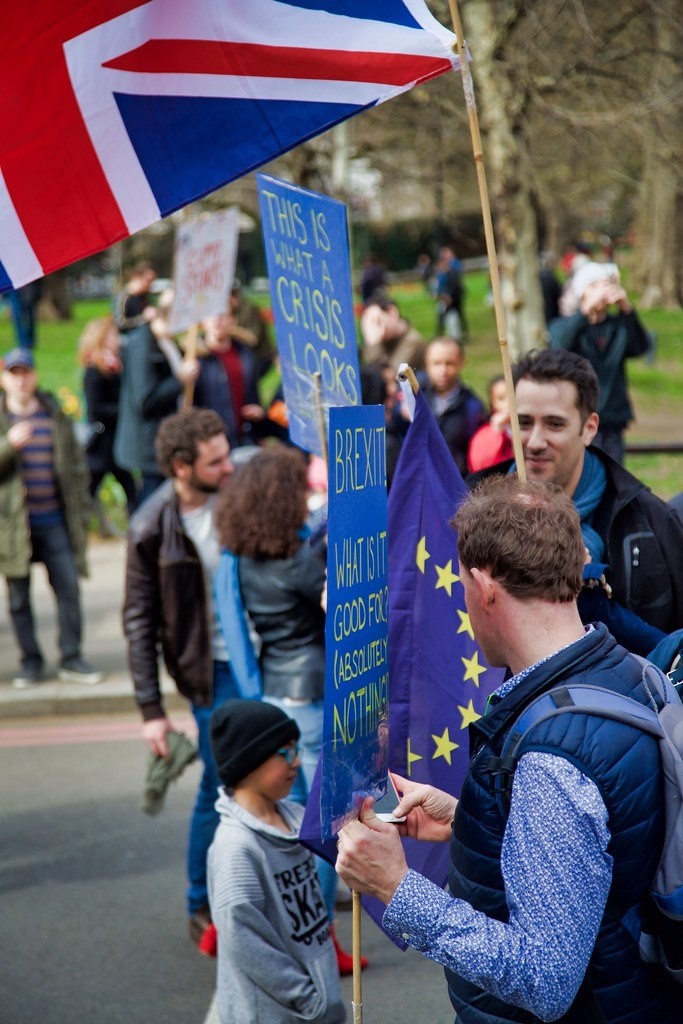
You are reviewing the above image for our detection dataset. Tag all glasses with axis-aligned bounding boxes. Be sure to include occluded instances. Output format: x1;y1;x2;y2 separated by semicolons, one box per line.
275;744;306;765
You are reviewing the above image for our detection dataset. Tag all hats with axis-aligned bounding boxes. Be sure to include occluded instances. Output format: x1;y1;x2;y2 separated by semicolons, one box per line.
5;347;34;372
210;701;302;788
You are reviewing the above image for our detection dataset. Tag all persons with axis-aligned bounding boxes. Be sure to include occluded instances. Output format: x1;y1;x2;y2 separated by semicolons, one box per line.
205;700;348;1023
0;248;683;972
334;476;682;1023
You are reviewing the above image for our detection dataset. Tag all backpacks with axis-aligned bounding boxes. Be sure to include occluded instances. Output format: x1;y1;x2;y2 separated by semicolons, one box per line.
486;651;683;985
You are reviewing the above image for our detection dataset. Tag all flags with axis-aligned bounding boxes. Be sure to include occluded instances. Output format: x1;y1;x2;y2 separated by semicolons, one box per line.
0;1;472;290
298;389;506;953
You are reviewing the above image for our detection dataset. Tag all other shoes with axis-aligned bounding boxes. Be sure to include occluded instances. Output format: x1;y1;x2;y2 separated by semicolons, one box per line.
189;909;212;944
12;659;44;689
57;653;100;683
329;924;368;974
